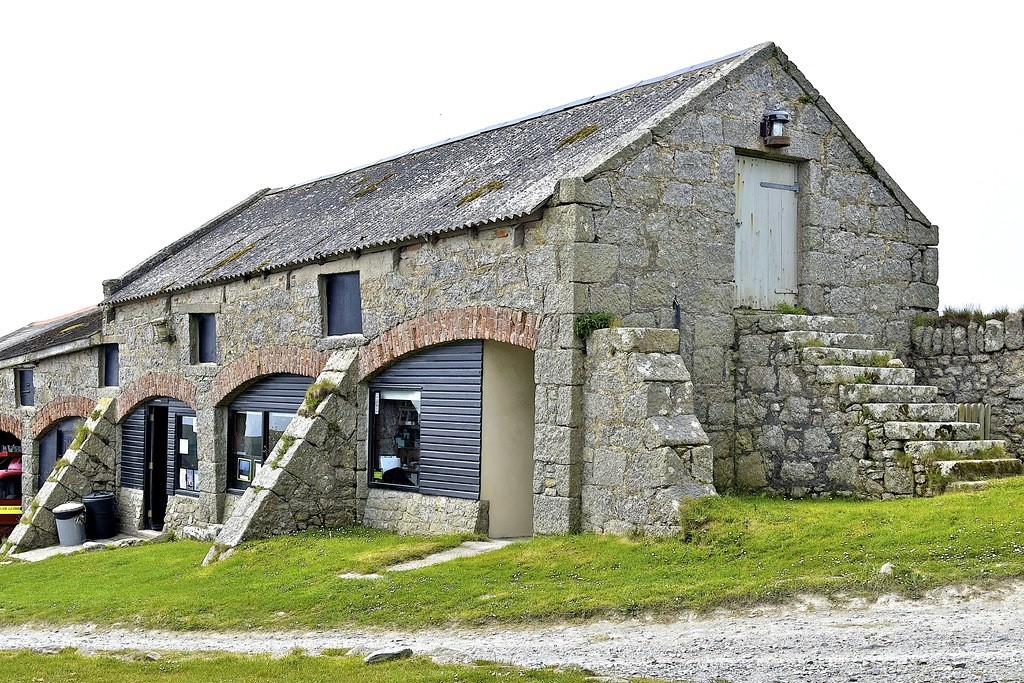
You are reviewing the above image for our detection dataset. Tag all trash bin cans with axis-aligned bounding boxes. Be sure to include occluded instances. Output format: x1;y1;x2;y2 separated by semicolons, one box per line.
51;502;86;547
81;491;117;541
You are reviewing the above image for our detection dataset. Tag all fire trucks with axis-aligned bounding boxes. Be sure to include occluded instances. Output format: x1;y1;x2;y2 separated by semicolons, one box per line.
0;446;24;541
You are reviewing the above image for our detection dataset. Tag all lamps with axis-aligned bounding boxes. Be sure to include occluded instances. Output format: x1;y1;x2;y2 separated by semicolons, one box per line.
763;111;791;146
150;318;176;344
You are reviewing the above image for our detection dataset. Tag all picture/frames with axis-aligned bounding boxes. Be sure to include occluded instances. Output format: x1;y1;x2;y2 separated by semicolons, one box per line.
237;457;252;482
